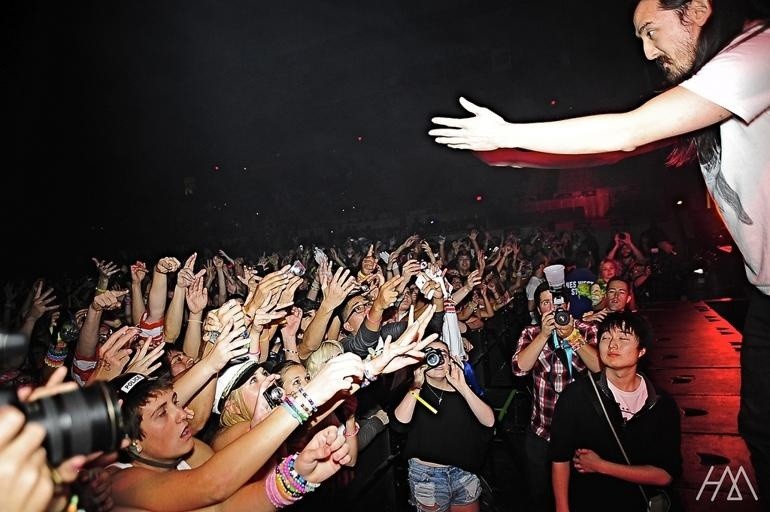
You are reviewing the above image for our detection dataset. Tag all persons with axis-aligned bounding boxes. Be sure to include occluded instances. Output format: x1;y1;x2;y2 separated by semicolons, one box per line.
429;0;770;512
1;215;728;512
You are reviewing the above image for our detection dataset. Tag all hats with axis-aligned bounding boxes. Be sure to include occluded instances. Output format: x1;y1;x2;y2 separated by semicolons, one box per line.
212;353;261;415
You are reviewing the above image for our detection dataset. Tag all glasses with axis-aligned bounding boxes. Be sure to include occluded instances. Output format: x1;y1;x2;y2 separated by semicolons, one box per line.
345;301;373;322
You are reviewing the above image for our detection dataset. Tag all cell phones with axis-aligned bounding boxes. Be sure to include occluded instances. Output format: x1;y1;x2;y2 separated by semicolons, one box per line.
253;265;264;283
414;275;435;300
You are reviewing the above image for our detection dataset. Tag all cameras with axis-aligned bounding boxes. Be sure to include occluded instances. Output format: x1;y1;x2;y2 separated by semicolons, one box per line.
413;259;429;271
0;330;120;467
618;231;627;240
285;260;306;280
550;283;572;330
263;384;286;409
359;279;372;294
420;347;444;371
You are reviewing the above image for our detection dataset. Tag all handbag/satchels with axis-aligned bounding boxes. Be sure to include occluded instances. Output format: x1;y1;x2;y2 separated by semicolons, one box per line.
645;490;672;512
